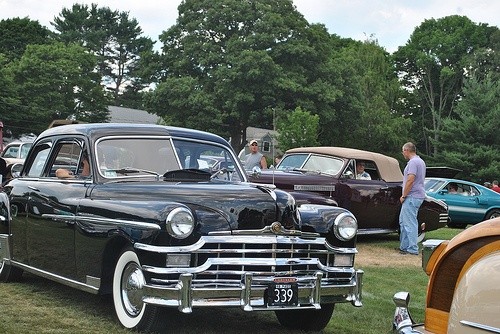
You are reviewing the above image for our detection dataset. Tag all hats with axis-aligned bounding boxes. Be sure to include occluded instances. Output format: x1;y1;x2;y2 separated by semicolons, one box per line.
249;140;258;145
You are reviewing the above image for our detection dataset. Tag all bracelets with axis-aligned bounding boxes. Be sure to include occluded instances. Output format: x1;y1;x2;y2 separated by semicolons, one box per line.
402;195;407;198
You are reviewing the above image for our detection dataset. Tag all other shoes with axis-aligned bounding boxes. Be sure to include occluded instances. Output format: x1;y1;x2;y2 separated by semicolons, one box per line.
394;247;407;254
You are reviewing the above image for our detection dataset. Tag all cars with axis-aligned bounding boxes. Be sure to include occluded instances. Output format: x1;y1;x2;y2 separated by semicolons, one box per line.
388;218;497;331
186;153;253;184
421;175;497;226
0;120;366;332
3;141;78;174
239;144;449;242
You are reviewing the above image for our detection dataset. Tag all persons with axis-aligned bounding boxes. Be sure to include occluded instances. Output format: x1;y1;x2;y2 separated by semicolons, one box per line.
398;142;426;255
449;182;458;193
484;180;500;193
55;148;104;178
356;162;372;180
244;139;267;171
274;154;283;165
